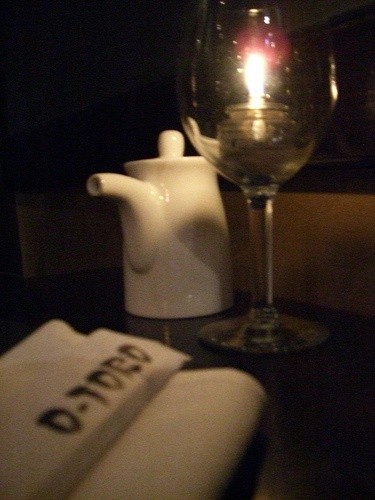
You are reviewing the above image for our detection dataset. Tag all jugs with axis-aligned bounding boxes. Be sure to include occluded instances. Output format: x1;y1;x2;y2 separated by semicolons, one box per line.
82;128;236;319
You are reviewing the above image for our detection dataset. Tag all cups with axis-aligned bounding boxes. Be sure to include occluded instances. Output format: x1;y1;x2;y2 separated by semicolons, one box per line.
180;0;338;353
